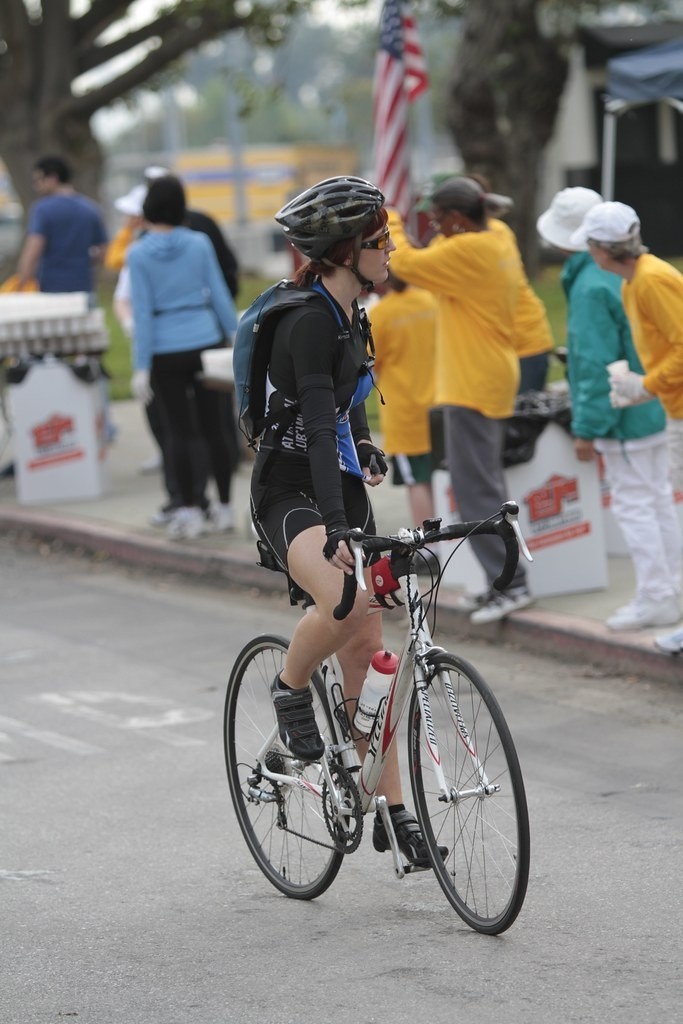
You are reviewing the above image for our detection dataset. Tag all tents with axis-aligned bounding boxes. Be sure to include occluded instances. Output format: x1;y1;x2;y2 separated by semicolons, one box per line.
601;41;683;201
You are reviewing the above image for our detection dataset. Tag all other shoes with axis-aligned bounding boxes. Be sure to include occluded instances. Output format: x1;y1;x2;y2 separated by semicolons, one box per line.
167;508;203;541
208;504;234;532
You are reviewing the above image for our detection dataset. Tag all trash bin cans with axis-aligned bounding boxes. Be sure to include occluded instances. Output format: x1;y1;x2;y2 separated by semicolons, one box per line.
427;389;610;599
2;354;110;504
591;451;683;559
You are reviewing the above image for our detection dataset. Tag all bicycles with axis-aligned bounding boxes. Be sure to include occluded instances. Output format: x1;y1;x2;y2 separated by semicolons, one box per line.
222;500;531;937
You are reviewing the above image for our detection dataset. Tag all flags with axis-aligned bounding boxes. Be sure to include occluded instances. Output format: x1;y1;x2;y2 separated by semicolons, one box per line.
374;0;429;230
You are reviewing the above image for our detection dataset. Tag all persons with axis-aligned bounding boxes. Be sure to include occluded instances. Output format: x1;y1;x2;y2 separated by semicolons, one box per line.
365;270;441;578
383;178;531;622
104;168;238;537
535;186;680;628
251;175;449;867
12;155;115;442
574;202;683;653
413;173;551;393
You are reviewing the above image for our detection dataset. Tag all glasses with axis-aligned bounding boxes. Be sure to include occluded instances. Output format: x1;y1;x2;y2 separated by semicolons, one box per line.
361;231;390;249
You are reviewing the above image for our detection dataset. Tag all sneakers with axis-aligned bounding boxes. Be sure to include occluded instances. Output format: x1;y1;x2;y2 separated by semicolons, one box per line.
372;811;449;867
604;595;681;628
469;590;532;623
270;668;325;759
654;627;683;654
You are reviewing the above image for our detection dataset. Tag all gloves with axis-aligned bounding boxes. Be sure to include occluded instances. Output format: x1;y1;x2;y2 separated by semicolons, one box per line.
607;372;654;408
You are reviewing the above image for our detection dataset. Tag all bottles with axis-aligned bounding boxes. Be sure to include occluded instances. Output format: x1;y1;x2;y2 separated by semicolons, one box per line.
352;650;398;736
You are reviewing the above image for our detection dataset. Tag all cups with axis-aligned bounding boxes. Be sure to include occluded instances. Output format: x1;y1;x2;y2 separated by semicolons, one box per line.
607;360;629;378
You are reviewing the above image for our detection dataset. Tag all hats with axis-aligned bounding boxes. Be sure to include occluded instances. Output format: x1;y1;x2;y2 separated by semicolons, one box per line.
571;202;640;246
536;187;605;252
142;178;184;225
115;185;149;216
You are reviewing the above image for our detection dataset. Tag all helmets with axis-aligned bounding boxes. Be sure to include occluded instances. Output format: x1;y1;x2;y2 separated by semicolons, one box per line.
273;176;385;259
